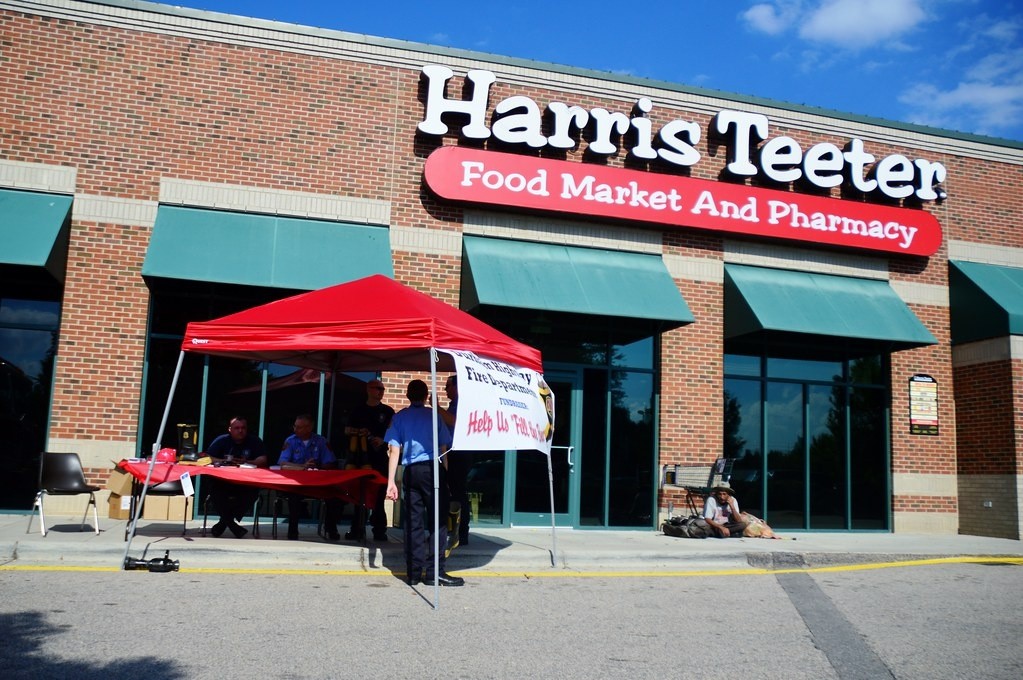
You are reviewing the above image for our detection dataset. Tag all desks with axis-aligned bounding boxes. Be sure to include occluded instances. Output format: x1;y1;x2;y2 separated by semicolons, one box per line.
114;458;390;547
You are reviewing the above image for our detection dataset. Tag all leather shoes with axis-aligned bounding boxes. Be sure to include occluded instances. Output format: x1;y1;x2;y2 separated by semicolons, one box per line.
426;571;464;586
408;576;420;585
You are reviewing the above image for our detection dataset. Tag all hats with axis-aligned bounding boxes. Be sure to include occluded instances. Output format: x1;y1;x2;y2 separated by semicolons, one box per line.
711;481;735;495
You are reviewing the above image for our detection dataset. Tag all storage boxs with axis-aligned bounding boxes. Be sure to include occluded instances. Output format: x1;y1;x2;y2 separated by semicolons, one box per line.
169;495;194;521
108;492;131;520
107;458;133;496
144;495;169;520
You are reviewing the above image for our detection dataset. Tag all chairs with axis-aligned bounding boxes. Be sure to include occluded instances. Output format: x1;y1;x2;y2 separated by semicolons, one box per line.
26;451;101;537
202;494;260;539
272;496;326;540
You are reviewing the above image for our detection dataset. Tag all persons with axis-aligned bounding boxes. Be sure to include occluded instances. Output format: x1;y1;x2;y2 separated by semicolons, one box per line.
345;380;395;543
428;375;470;545
206;416;268;537
277;415;341;540
383;380;463;587
703;481;741;537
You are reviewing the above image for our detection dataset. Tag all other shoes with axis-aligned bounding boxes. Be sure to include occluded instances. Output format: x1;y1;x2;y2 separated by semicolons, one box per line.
373;533;387;541
230;522;248;538
211;521;227;535
323;523;341;540
345;530;366;540
288;523;298;540
460;537;469;544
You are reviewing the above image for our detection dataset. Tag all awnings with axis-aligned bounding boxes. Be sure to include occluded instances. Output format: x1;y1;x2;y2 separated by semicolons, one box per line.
460;235;696;323
0;187;74;283
141;205;395;290
724;262;939;345
948;259;1023;347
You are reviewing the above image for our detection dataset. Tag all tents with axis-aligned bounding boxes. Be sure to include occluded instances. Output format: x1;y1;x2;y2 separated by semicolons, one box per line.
120;274;557;609
233;367;367;395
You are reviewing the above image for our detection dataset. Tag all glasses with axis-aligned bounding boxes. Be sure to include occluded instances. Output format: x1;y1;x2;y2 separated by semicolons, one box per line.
371;386;385;391
